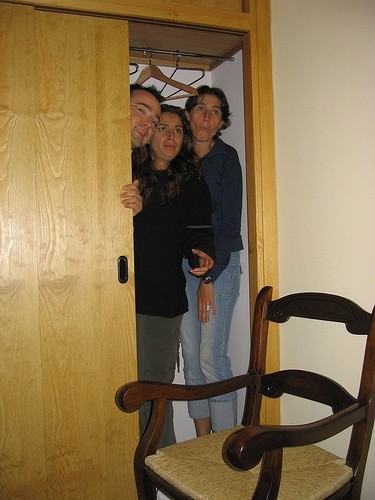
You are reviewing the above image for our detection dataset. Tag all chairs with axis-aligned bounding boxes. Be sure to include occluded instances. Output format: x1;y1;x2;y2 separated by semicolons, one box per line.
114;286;375;500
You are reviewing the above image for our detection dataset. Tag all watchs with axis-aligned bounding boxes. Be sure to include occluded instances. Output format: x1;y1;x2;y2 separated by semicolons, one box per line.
202;274;214;284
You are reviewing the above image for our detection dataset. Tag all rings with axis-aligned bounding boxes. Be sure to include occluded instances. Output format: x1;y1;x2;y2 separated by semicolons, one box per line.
206;304;211;311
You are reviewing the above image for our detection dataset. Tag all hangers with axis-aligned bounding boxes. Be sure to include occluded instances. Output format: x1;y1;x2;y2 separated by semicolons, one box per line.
135;46;198;102
130;62;139;76
161;51;205;98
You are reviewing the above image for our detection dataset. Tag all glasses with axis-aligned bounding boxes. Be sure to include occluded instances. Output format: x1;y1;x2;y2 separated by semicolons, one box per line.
130;102;160;129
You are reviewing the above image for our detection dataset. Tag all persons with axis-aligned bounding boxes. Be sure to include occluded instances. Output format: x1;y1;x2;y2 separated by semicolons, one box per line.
120;83;167;218
178;86;243;438
134;104;216;449
